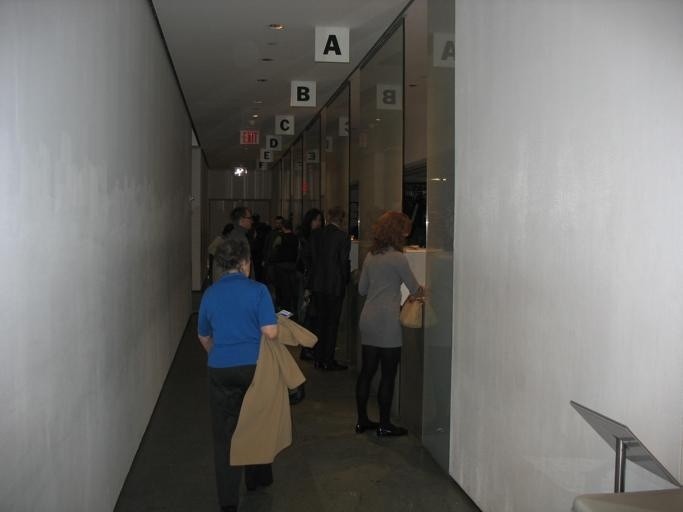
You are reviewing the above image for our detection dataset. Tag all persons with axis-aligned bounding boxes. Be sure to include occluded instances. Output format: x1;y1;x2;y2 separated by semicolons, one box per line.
206;206;351;371
197;235;281;511
354;210;424;438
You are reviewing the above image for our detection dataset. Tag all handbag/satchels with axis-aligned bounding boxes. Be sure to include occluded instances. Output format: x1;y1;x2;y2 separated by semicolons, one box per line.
400;295;422;329
201;275;212;292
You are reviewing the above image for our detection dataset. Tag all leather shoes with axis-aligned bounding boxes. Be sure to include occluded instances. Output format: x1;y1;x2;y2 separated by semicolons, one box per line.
299;352;313;361
356;422;378;433
314;360;347;371
378;424;407;437
